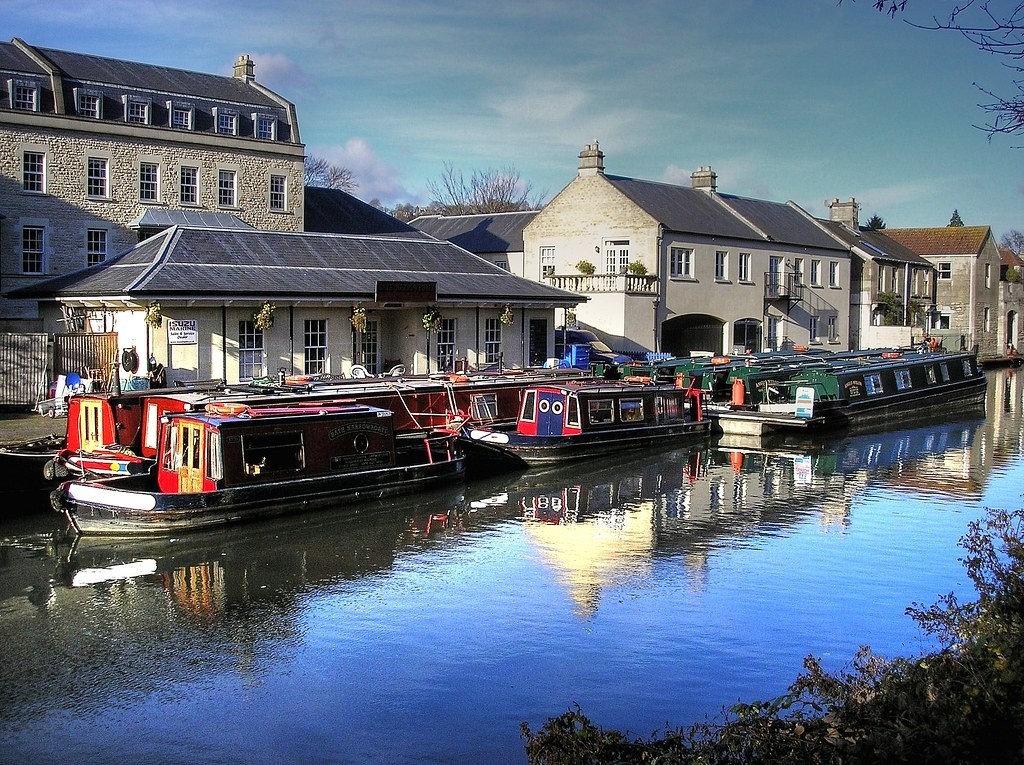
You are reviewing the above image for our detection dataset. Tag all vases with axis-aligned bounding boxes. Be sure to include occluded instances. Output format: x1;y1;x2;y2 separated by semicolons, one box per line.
620;267;628;274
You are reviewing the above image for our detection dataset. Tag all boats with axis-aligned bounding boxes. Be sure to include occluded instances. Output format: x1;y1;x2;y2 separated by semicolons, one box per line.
590;351;986;431
457;377;714;469
44;384;472;536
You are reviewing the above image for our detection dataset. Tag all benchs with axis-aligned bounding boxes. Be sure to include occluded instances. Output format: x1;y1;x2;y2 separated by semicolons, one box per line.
310;372;346;381
172;378;225;386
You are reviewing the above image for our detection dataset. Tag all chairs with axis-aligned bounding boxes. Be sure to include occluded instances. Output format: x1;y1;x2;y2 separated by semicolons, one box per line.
350;364;375;379
382;364;406;377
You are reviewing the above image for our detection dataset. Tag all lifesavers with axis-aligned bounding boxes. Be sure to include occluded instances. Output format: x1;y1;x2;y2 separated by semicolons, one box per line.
450;375;469;383
204;401;247;413
882;352;899;358
624;374;651;382
285;375;309;385
793;345;808;351
711;357;729;364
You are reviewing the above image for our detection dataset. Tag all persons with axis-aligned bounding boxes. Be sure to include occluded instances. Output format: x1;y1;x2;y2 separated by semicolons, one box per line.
926;338;938;350
746;339;753;353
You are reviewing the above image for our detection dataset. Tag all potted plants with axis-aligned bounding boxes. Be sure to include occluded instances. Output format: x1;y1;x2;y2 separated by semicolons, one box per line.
421;307;443;333
497;304;515;325
575;260;596;274
628;260;649;275
252;301;277;331
143;302;162;329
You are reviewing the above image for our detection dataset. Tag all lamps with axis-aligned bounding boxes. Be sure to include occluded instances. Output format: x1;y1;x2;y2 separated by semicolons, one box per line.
787;259;790;265
595;246;599;253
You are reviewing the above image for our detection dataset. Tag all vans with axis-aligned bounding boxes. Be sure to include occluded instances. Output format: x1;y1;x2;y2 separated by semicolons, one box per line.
555;328;633;368
